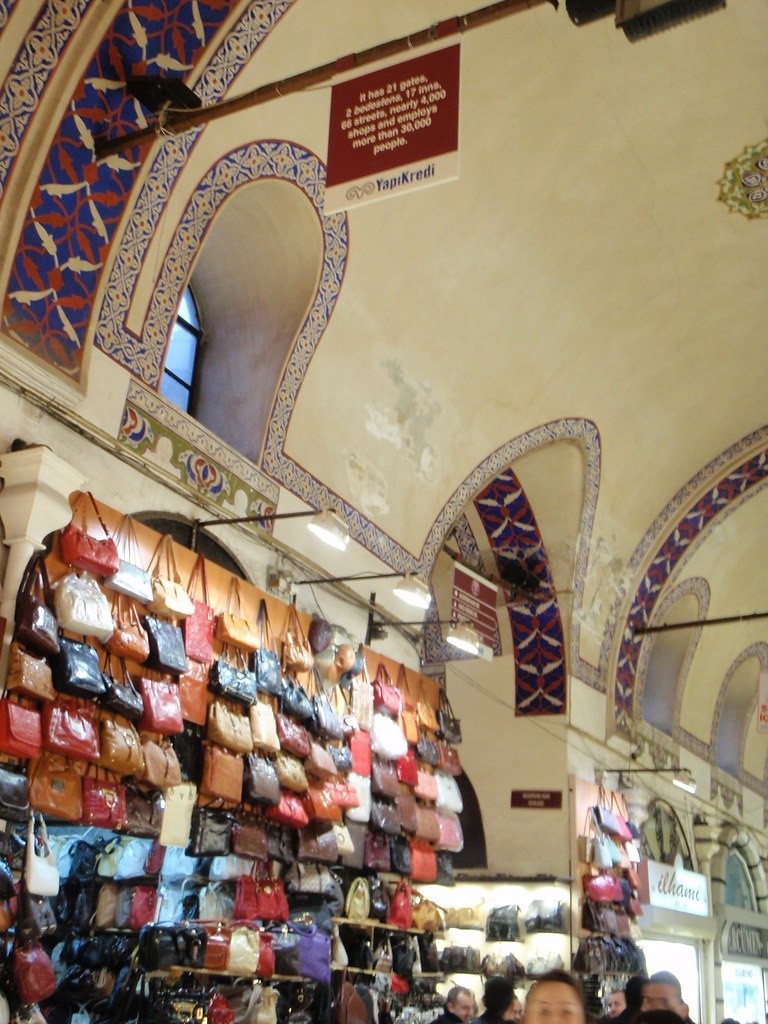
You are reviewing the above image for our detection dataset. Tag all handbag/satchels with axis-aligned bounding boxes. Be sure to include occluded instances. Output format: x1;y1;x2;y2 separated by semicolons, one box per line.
0;488;563;1024
577;785;643;978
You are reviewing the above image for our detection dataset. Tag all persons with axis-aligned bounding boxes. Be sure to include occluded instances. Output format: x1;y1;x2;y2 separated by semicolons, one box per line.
720;1018;760;1024
523;968;586;1024
428;987;475;1024
596;970;696;1024
471;974;523;1024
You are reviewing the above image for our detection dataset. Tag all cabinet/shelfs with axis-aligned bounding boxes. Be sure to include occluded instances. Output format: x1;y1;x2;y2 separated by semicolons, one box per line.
332;915;445;978
412;872;572;980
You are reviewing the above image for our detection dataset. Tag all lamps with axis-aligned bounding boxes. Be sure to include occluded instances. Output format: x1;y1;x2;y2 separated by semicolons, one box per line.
605;767;697;794
197;508;350;552
292;569;431;610
364;593;479;656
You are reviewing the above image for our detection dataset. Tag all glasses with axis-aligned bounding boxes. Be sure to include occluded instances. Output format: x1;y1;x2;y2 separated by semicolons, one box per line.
643;995;670;1010
